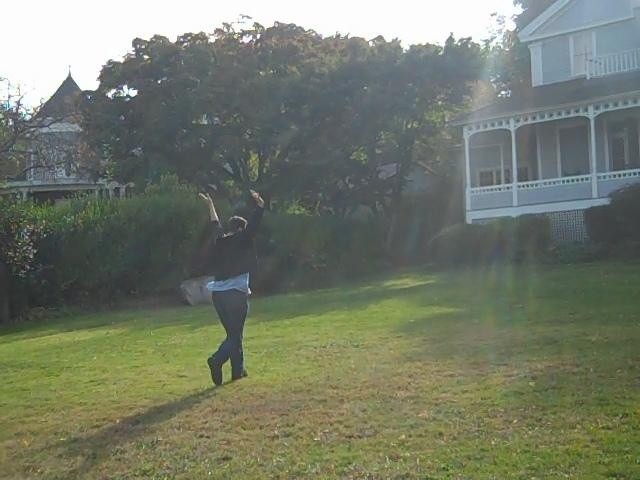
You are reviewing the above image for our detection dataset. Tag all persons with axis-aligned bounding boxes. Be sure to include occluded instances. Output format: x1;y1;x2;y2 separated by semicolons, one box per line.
197;190;267;388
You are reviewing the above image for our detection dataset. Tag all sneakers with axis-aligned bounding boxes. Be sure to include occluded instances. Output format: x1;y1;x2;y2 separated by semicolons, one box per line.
232;369;247;380
208;355;223;386
